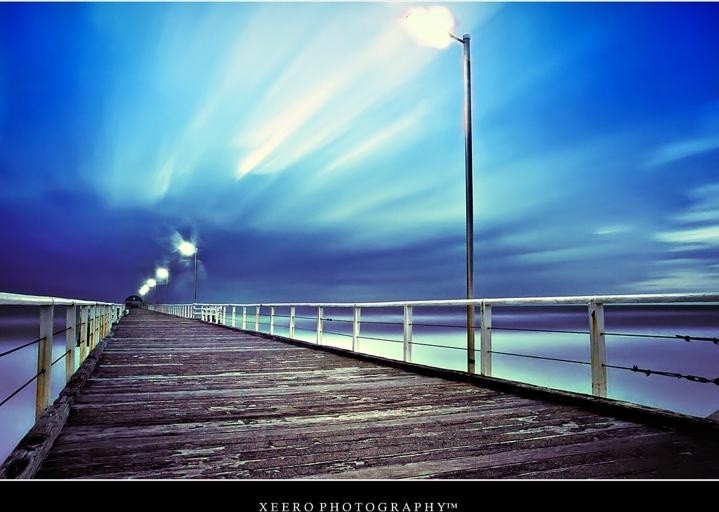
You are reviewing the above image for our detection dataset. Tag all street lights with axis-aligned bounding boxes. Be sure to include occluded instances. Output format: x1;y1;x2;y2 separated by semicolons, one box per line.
182;244;198;304
158;268;168;304
401;4;477;377
